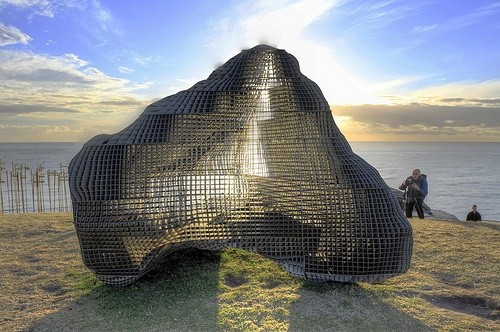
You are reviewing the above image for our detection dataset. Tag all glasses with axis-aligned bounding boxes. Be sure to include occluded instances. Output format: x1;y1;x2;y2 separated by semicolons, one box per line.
412;172;420;175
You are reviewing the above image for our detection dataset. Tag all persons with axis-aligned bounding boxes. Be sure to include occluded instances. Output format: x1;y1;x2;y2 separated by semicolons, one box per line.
400;168;428;219
466;205;481;221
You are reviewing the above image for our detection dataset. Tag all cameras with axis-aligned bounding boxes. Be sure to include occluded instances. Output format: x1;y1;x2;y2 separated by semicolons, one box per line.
405;179;412;187
